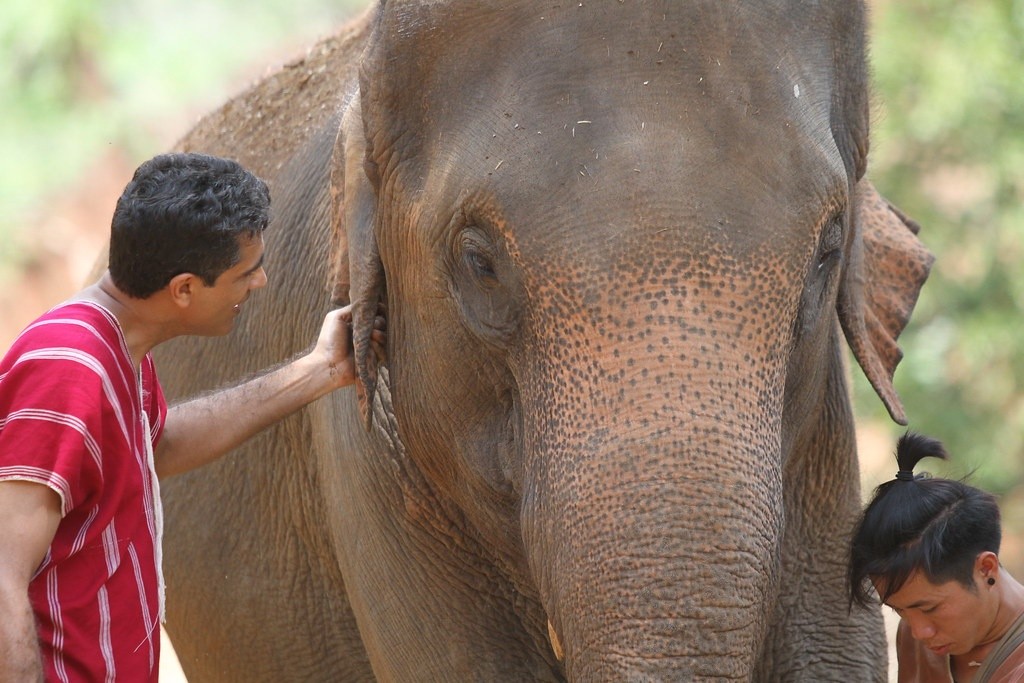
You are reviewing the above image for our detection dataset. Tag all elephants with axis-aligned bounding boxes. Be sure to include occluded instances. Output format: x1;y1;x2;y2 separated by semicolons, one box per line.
159;0;932;682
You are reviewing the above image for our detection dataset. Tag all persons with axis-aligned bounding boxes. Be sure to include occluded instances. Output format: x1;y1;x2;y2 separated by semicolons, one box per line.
846;432;1024;683
0;153;390;683
